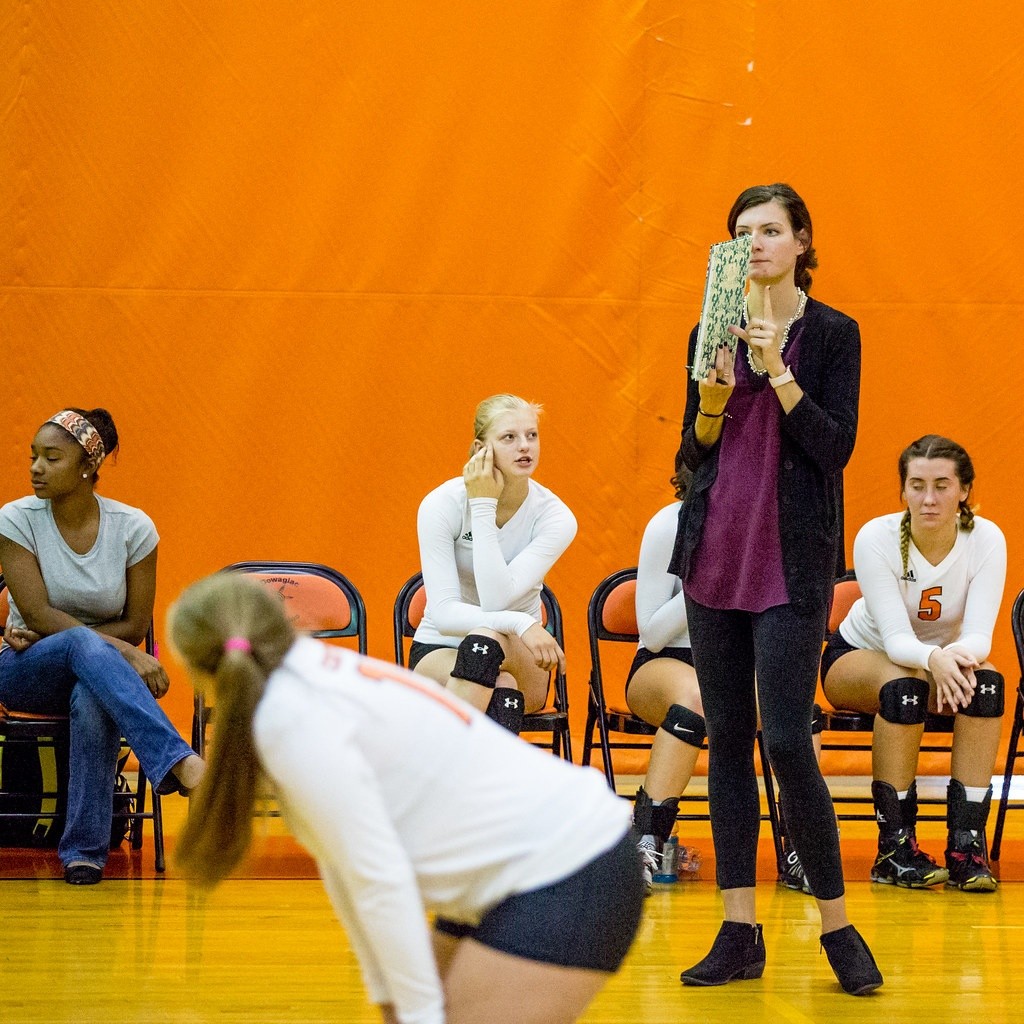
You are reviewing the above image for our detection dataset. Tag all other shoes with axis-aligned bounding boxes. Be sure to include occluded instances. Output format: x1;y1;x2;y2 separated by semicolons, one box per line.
179;783;191;798
64;861;102;886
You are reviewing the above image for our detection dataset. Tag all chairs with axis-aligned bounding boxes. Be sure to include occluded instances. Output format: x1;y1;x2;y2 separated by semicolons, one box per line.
784;569;989;868
0;572;166;873
991;588;1024;860
582;567;784;872
393;570;572;765
188;560;367;817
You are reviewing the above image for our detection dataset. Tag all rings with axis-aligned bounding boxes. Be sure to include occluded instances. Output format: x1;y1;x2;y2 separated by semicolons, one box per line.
724;373;729;376
759;320;768;329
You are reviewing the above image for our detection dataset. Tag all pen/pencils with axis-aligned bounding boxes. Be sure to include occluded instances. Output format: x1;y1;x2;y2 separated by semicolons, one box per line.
685;365;728;385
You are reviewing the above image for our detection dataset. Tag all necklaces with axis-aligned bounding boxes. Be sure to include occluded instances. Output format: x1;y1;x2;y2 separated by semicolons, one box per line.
743;291;807;375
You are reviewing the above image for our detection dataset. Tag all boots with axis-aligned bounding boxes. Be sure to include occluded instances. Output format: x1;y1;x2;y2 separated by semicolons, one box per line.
781;853;811;893
943;779;998;891
870;781;949;887
633;784;680;892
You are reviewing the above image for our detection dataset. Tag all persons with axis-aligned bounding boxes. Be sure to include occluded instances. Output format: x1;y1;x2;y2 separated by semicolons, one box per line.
0;405;207;885
165;574;648;1024
625;501;812;894
674;181;884;995
407;391;577;735
820;434;1007;893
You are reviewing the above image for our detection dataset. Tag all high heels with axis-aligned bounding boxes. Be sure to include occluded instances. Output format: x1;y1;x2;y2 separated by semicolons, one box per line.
680;921;767;985
820;924;883;995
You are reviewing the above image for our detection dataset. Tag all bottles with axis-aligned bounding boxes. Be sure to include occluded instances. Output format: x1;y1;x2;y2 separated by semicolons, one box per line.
652;819;679;883
678;844;704;872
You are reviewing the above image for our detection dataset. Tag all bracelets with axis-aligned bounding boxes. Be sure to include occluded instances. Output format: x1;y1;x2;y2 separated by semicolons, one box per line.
698;408;724;417
768;365;796;388
